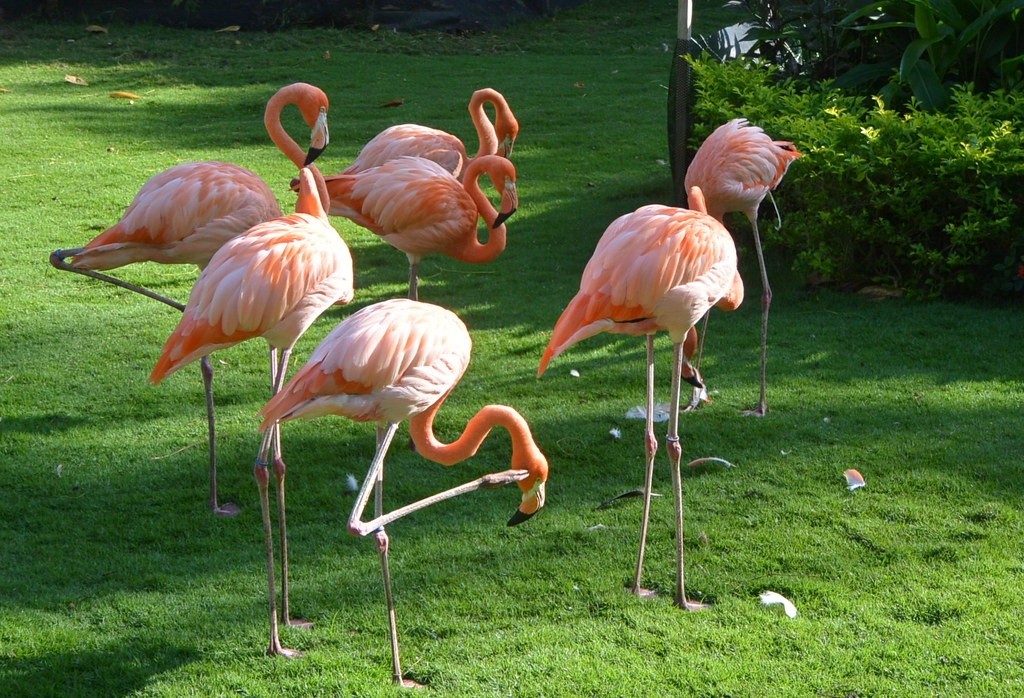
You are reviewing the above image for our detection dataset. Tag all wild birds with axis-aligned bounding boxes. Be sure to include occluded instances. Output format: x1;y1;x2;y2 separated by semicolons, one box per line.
48;81;356;664
287;87;521;302
534;115;802;616
253;295;550;691
